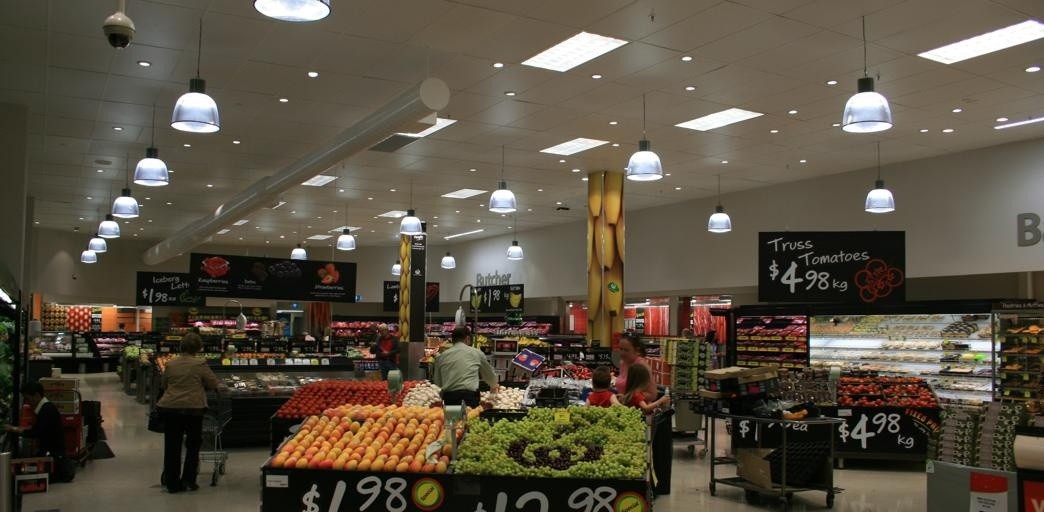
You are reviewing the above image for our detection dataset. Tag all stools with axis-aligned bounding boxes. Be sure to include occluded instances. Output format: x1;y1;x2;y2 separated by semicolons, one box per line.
54;455;64;462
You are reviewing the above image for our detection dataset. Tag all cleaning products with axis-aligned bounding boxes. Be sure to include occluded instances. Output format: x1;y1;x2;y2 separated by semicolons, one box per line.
699;412;846;510
40;388;96;483
656;386;703;457
181;385;235;489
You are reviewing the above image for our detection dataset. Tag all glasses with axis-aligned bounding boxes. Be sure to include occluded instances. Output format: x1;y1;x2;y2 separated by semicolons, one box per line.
399;179;422;236
391;259;401;276
289;243;307;261
625;92;664;183
255;0;335;23
506;214;524;261
864;142;896;214
489;145;517;214
840;15;894;133
708;175;732;234
80;16;221;265
336;205;356;251
441;252;457;270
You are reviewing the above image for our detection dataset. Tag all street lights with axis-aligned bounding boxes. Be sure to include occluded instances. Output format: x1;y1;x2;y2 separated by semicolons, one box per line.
147;406;166;434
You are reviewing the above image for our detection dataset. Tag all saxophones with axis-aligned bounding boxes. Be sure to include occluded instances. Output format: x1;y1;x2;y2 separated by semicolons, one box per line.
480;386;525;409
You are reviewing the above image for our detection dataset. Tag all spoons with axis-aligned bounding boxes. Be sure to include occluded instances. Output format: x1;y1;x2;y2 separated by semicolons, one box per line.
276;380;442;419
837;376;936;408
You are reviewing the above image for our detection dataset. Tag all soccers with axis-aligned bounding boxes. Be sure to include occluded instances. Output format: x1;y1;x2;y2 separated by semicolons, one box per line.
271;404;465;473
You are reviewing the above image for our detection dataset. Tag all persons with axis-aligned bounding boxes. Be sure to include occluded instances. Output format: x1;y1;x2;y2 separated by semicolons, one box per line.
430;327;499;406
377;324;400;379
616;332;658;420
707;329;726;371
624;363;666;410
585;366;624;408
118;323;129;334
5;381;66;483
389;326;399;336
156;331;218;493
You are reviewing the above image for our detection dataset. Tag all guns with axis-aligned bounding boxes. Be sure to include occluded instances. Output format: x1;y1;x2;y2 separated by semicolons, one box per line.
588;171;624;348
472;335;487;349
517;336;547;347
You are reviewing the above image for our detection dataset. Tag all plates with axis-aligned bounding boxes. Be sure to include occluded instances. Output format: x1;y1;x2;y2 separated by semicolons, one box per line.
727;298;1043;413
1;293;135;465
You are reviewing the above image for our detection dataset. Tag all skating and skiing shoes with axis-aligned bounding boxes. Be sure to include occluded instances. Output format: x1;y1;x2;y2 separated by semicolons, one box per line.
453;403;648;478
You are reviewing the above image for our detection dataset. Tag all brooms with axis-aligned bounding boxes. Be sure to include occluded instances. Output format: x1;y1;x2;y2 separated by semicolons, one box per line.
400;235;411;342
